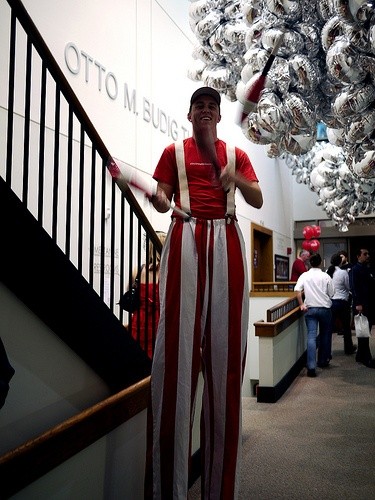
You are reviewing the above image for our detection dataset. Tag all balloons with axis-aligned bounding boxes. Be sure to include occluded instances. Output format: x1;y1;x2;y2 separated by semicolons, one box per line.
188;0;375;232
302;224;321;252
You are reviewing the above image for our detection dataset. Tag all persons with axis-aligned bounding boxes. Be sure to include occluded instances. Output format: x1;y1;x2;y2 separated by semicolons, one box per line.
290;247;310;282
143;86;263;500
349;245;375;368
294;253;334;377
125;231;167;360
324;250;357;356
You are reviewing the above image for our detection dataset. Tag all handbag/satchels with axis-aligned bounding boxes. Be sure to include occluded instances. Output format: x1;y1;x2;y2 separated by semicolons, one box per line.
354;313;370;338
118;264;146;313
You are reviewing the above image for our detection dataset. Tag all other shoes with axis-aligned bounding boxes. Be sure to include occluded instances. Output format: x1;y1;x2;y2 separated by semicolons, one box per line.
307;369;317;377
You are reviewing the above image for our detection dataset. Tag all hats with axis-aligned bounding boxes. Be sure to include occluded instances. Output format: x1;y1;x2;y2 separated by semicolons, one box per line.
190;87;221;105
